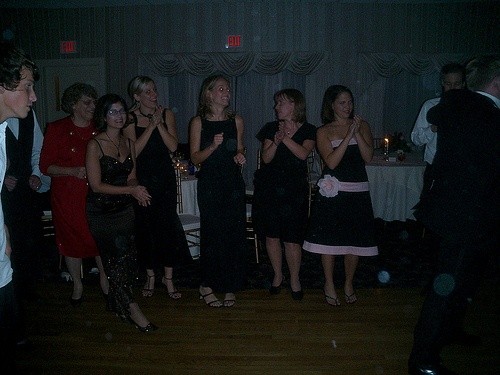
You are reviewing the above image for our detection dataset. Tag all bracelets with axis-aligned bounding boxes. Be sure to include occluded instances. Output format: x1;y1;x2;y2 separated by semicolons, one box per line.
273;141;278;147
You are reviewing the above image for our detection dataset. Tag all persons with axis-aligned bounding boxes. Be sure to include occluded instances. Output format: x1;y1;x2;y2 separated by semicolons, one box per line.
38;83;113;309
123;76;195;299
411;63;466;199
0;40;52;338
256;89;317;302
409;54;500;375
189;75;247;308
302;85;379;306
85;93;157;333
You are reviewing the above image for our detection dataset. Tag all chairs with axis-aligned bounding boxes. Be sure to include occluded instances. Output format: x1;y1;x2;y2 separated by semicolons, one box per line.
255;148;311;263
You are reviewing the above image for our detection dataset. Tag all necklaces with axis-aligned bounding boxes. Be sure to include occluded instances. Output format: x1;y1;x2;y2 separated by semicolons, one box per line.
105;131;121;157
285;126;296;134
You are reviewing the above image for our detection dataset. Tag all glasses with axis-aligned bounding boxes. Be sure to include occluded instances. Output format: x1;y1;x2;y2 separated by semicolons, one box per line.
79;98;98;106
107;107;129;116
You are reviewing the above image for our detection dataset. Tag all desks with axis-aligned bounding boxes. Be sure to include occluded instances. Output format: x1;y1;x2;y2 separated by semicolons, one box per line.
175;174;203;259
365;146;432;223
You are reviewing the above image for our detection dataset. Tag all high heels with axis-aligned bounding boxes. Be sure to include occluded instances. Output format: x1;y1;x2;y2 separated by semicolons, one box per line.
198;290;223;307
270;280;284;294
72;283;85;307
223;294;237;308
99;283;110;299
344;289;357;305
115;311;128;322
161;275;182;299
289;284;303;301
322;286;341;307
143;273;155;296
127;315;156;332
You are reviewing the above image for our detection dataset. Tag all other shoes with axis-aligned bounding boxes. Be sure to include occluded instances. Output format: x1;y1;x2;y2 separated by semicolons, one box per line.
448;331;482;347
408;362;457;375
13;287;36;301
82;273;100;285
